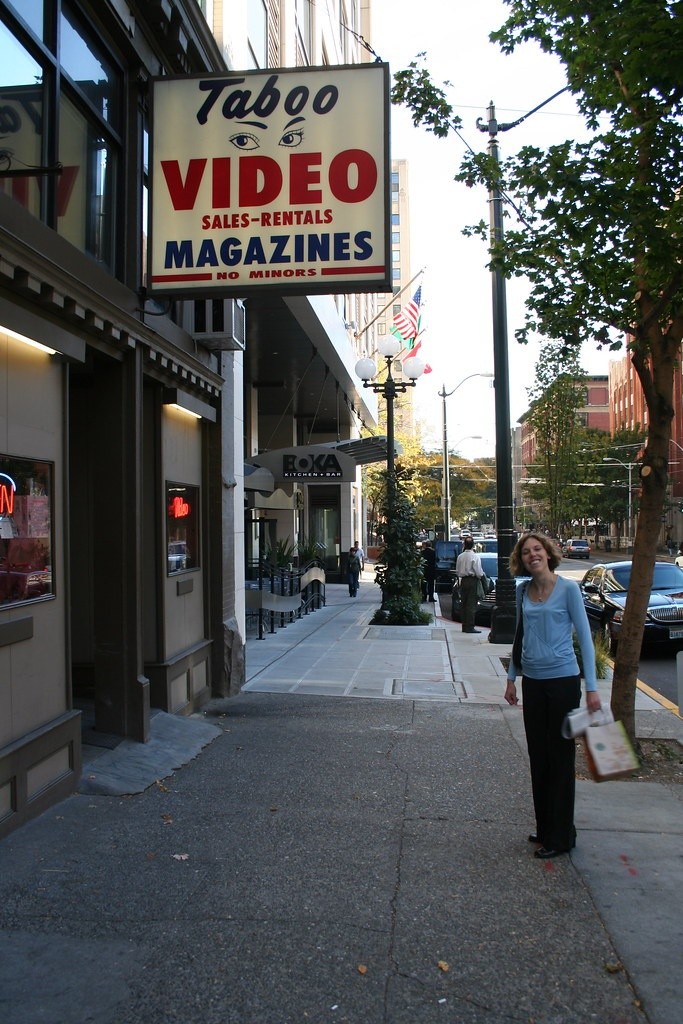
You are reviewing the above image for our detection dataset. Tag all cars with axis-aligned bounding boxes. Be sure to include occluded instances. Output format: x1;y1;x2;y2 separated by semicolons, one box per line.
577;561;683;659
449;552;534;623
432;528;499;594
562;539;590;559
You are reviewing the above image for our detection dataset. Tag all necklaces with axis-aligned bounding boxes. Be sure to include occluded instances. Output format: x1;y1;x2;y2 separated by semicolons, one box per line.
538;596;542;602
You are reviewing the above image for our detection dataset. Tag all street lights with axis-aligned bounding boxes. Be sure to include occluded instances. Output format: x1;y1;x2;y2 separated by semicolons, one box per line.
602;456;633;542
436;374;499;536
353;334;425;512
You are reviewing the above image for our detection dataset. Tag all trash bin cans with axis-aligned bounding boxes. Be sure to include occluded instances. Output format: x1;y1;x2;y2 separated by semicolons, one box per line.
604;539;611;552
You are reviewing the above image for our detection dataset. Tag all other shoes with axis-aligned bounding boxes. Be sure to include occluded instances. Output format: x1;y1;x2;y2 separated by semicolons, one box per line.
350;591;356;597
429;597;437;602
463;628;481;633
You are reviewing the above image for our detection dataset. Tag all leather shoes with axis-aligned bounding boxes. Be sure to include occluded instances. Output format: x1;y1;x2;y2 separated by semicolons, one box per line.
528;832;539;843
533;845;572;858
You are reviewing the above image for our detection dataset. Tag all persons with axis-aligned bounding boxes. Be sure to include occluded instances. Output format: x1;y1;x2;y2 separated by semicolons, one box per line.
349;541;364;591
344;548;361;597
456;537;483;633
665;537;673;557
504;532;601;858
417;539;438;603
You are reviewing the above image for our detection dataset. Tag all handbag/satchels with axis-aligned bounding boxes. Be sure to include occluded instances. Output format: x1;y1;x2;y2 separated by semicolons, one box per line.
512;581;529;658
585;708;640;782
347;563;352;571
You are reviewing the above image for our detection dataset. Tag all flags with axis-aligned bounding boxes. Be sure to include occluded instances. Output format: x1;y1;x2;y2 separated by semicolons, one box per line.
390;316;421;349
401;342;432;374
394;286;420;339
